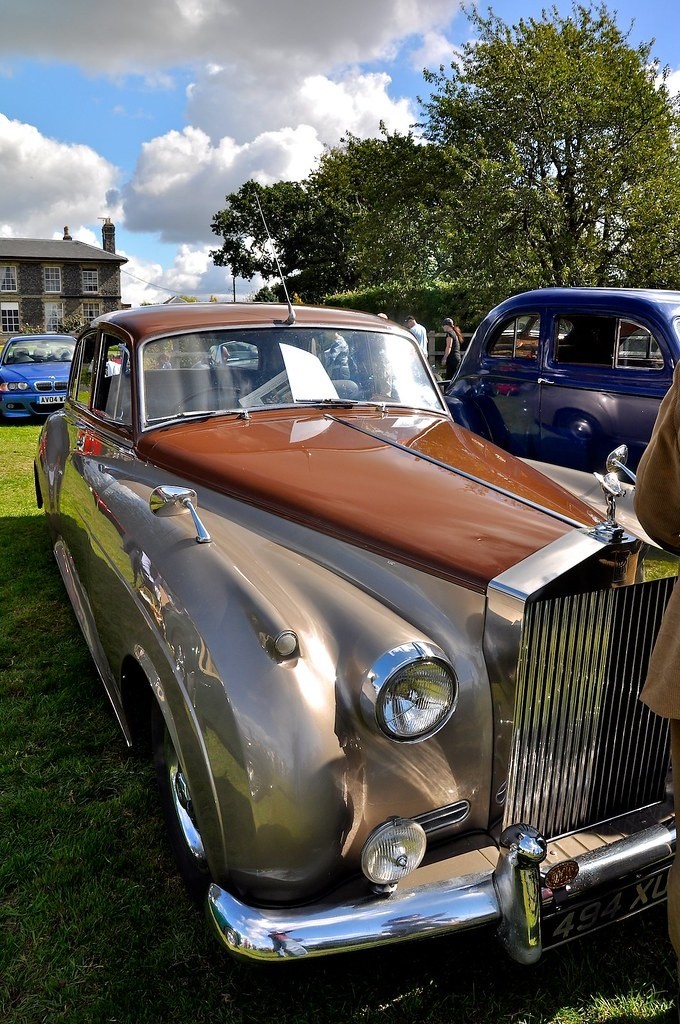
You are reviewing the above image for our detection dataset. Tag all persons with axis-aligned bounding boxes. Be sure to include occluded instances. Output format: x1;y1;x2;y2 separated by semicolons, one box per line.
634;358;680;1002
441;318;464;391
516;317;593;348
311;330;358;400
404;315;428;361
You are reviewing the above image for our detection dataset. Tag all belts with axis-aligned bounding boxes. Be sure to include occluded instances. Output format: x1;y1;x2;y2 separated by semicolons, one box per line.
332;365;349;369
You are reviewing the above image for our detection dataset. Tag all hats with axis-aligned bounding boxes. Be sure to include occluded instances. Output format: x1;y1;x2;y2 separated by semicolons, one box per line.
441;318;453;326
404;315;414;322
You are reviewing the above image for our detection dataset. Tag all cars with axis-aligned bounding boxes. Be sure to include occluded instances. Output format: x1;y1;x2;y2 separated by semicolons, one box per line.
189;341;259;372
2;333;83;425
33;300;679;973
440;284;679;500
